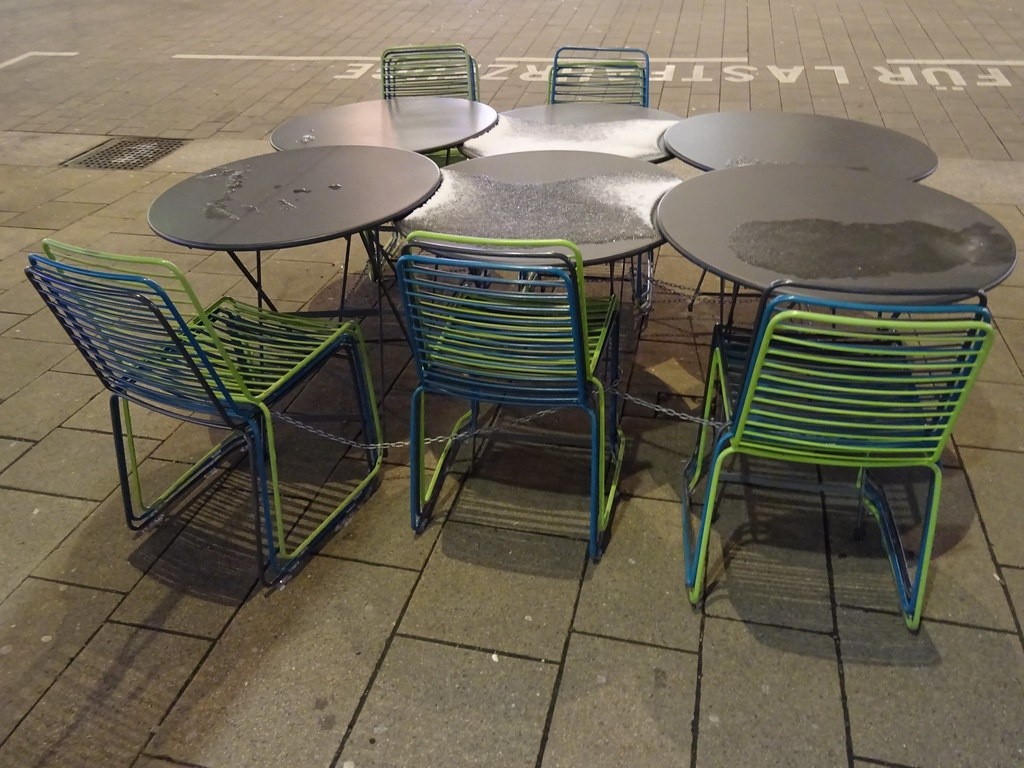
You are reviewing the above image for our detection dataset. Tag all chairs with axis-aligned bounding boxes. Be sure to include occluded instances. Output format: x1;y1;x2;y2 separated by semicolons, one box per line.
380;42;480;101
396;231;625;563
546;46;650;109
24;239;386;586
680;279;994;632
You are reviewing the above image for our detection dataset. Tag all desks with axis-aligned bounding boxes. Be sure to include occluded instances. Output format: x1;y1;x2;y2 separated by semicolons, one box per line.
148;97;1017;362
